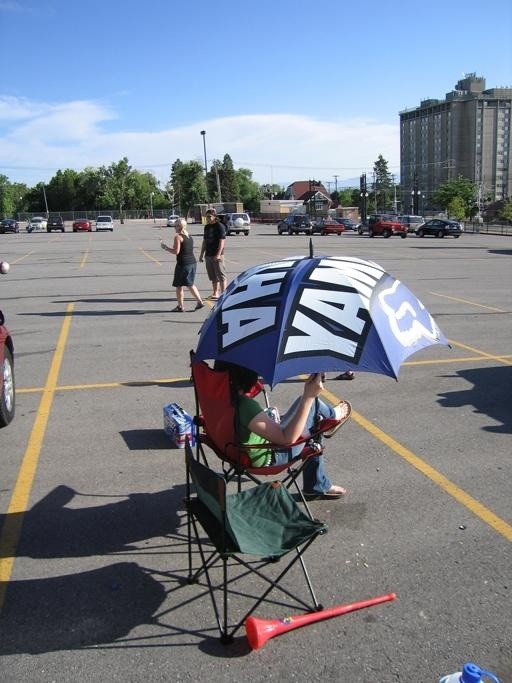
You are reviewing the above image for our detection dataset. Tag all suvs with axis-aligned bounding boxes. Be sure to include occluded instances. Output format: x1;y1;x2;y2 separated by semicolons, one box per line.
368;213;408;238
47;215;65;233
223;212;251;235
278;213;312;235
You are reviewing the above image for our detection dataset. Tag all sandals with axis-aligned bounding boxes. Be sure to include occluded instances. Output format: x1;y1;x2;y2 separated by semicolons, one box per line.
171;304;184;311
195;302;205;308
336;372;354;379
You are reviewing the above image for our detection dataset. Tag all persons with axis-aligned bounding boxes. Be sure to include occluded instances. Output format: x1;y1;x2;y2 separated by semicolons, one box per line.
213;359;353;500
334;370;356;380
160;216;207;313
197;208;228;301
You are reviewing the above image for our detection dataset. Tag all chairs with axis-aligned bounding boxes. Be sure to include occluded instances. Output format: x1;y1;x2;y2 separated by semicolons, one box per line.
177;431;333;650
186;344;343;528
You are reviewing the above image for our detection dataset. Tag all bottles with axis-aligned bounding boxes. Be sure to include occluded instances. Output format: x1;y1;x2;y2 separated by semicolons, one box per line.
441;662;499;682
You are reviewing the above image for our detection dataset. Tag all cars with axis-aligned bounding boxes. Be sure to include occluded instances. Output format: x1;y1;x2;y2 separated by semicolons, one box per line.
358;219;369;234
335;217;355;229
27;216;47;233
73;218;93;231
0;218;20;233
313;219;345;236
166;214;179;227
0;310;16;426
416;218;461;238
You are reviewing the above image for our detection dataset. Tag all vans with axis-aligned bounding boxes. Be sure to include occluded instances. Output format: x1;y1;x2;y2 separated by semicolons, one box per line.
96;215;114;231
400;214;425;232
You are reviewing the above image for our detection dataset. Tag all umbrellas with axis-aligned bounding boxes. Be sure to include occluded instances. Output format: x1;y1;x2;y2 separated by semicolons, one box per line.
189;234;454;451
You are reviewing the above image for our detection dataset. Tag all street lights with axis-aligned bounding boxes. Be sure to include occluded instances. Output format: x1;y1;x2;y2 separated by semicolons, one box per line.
150;192;155;216
411;171;421;215
360;173;369;220
200;129;210;188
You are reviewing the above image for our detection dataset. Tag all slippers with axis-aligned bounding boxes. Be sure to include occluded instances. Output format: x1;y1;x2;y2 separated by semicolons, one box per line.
301;485;344;500
208;295;219;301
322;399;352;438
